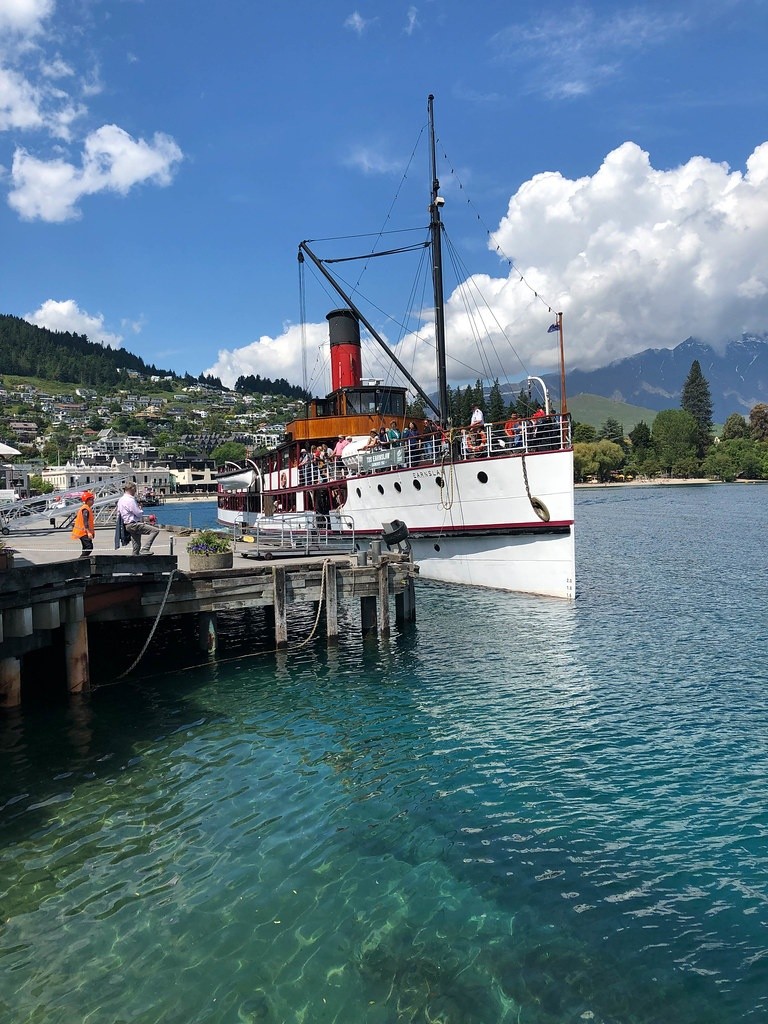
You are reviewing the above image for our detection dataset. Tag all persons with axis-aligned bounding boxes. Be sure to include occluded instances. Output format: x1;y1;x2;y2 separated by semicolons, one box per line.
72;493;95;558
299;402;561;485
117;482;159;556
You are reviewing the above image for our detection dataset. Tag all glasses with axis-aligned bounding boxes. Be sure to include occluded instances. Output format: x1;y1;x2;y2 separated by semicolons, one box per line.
423;422;427;425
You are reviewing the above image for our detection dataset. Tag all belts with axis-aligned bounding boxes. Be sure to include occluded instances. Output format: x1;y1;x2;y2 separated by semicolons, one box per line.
124;521;141;526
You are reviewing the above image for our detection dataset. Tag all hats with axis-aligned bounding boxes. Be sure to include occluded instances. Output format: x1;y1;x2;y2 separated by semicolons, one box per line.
300;449;308;453
471;403;478;407
389;420;398;427
370;427;379;436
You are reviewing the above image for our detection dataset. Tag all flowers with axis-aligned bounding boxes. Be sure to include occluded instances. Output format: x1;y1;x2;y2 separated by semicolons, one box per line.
185;531;230;557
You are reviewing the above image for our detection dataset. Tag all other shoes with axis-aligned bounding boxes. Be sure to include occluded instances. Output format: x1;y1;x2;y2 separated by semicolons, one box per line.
139;549;154;555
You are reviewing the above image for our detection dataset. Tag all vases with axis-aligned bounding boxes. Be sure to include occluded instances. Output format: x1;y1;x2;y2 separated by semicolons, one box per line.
189;549;233;571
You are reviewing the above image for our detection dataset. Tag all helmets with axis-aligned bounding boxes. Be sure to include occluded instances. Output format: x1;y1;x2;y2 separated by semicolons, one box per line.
81;492;94;502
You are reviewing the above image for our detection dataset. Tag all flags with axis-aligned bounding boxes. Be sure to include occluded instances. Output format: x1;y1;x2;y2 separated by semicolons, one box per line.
547;324;560;334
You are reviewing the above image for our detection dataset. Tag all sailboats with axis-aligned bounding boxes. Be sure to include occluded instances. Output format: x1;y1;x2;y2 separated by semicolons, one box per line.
216;95;576;599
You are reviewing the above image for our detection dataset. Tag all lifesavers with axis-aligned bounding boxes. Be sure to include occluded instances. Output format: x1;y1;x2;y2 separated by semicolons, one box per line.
467;430;486;451
281;473;286;487
532;497;551;522
